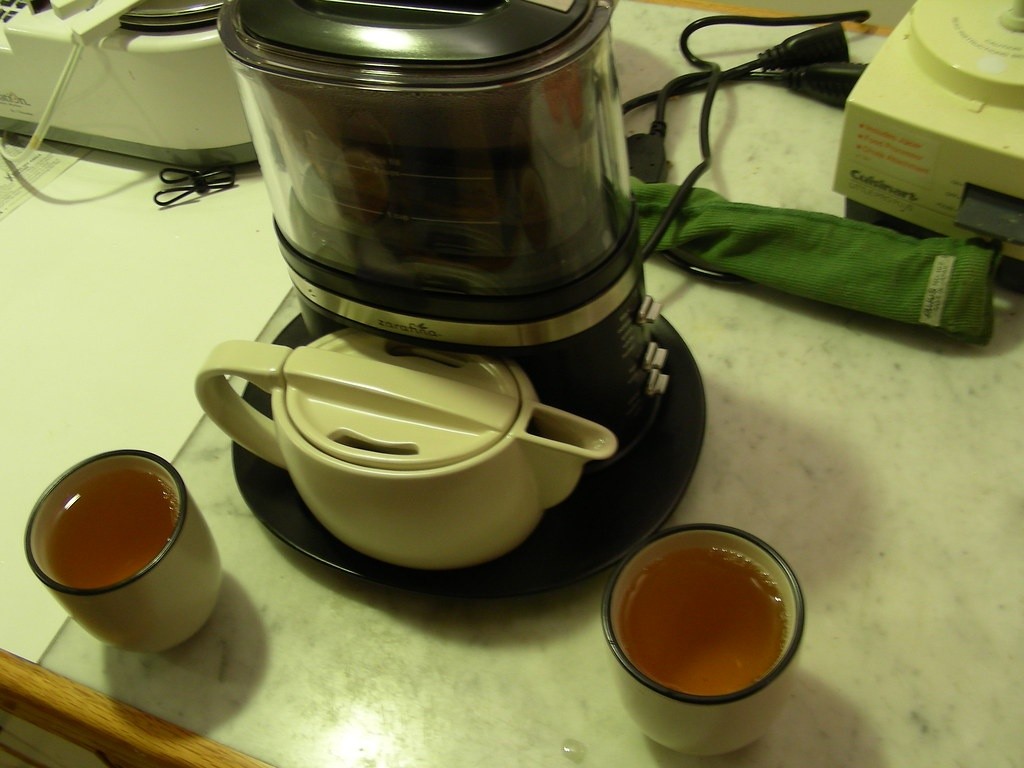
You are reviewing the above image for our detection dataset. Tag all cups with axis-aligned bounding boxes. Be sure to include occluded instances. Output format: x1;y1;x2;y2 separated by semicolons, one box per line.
24;449;222;657
602;522;803;755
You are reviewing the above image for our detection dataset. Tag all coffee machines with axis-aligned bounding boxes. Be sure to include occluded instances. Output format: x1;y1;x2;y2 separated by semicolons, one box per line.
214;0;668;481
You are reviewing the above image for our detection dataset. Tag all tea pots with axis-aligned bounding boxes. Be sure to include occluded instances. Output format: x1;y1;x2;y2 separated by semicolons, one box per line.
196;327;617;568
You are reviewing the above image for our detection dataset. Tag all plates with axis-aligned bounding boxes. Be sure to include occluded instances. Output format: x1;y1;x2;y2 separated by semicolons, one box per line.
232;305;708;596
